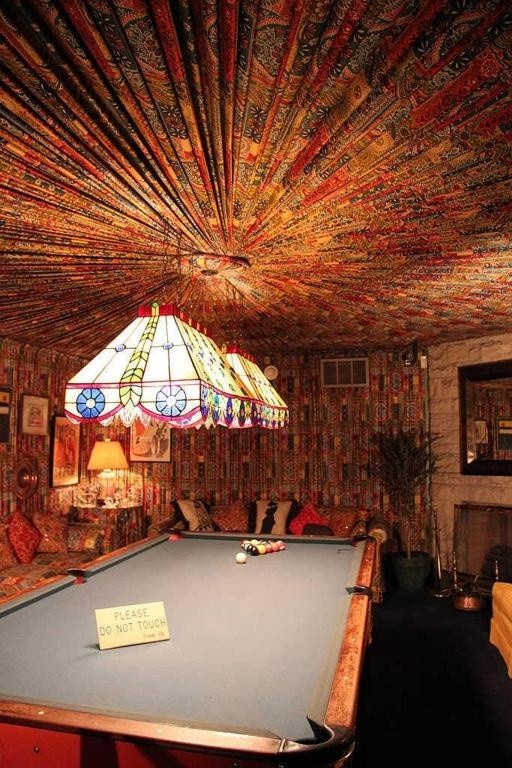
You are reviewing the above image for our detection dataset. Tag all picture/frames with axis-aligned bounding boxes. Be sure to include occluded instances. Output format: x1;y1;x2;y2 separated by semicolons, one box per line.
18;392;82;488
130;418;172;464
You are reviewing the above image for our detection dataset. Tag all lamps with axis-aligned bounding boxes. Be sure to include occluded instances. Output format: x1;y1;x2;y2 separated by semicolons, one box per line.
62;300;291;431
88;438;131;506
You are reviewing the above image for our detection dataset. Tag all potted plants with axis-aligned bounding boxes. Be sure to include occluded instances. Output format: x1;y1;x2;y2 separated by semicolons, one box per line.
363;421;451;598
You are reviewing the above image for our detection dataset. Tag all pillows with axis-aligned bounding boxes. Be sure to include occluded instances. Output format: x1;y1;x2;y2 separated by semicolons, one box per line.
170;498;359;536
1;513;106;571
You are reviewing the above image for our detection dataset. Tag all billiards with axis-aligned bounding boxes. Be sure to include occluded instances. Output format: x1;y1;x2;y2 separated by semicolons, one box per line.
236;539;286;563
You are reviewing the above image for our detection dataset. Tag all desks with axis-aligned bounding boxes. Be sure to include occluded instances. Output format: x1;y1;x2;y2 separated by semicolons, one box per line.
0;527;378;768
71;498;145;549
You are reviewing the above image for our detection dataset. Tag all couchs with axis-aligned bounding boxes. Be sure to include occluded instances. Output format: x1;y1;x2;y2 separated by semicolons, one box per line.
148;498;389;605
0;523;116;600
